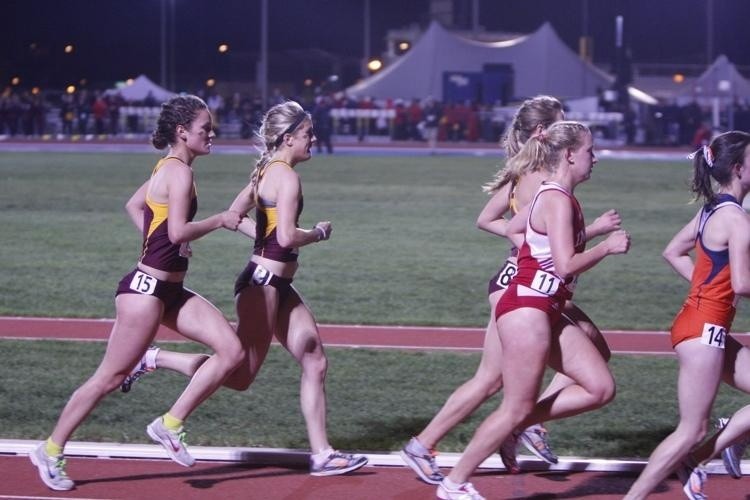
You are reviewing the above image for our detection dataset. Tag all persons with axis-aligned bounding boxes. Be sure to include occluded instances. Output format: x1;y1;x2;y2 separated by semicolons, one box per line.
121;102;368;478
0;73;750;156
434;122;630;500
401;96;612;484
622;129;750;499
29;95;246;492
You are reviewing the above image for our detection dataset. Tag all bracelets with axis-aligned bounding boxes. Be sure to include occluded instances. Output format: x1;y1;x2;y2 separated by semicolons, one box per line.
313;224;327;243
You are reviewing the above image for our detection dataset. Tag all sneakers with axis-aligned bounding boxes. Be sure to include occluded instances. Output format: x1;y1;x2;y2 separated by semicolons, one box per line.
399;438;444;485
518;426;559;464
714;418;745;480
436;481;485;500
309;452;368;477
28;442;75;491
146;416;195;467
120;346;156;392
499;432;521;473
675;457;708;499
